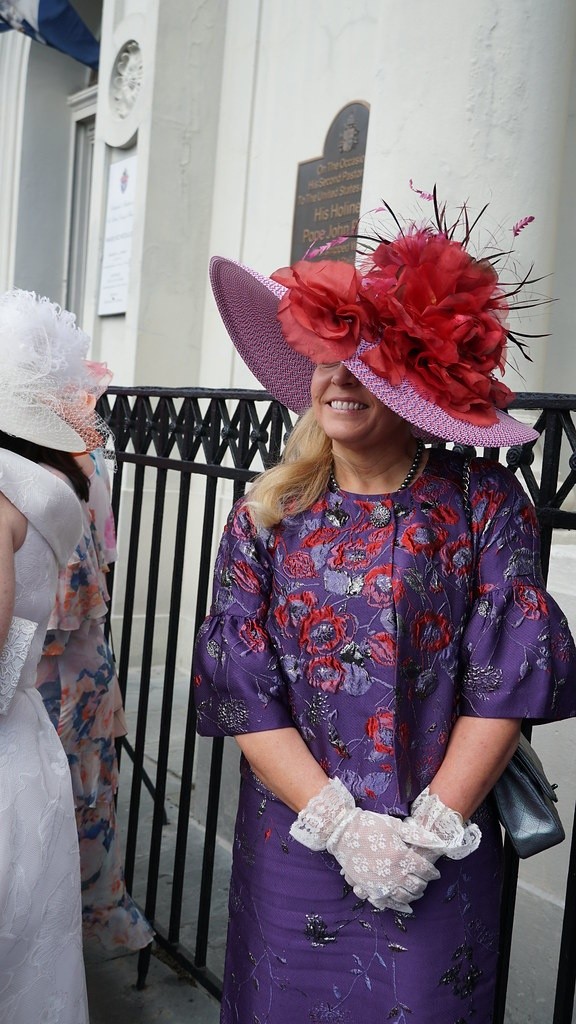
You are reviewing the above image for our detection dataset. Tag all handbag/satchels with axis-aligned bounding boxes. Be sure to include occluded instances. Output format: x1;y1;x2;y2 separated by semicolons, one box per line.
495;731;564;859
0;617;39;718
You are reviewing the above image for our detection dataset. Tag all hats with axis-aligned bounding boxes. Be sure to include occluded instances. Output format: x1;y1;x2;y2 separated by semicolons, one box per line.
0;290;119;475
207;179;559;447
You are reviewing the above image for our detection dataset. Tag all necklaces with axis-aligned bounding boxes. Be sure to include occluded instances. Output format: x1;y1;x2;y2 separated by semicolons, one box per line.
330;441;424;492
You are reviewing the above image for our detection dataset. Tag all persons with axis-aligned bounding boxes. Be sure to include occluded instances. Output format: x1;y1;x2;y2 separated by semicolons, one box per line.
48;361;154;953
0;282;100;1023
195;184;576;1024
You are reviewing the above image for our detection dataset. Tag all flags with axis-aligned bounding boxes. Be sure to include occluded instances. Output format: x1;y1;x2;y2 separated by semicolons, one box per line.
0;0;100;72
